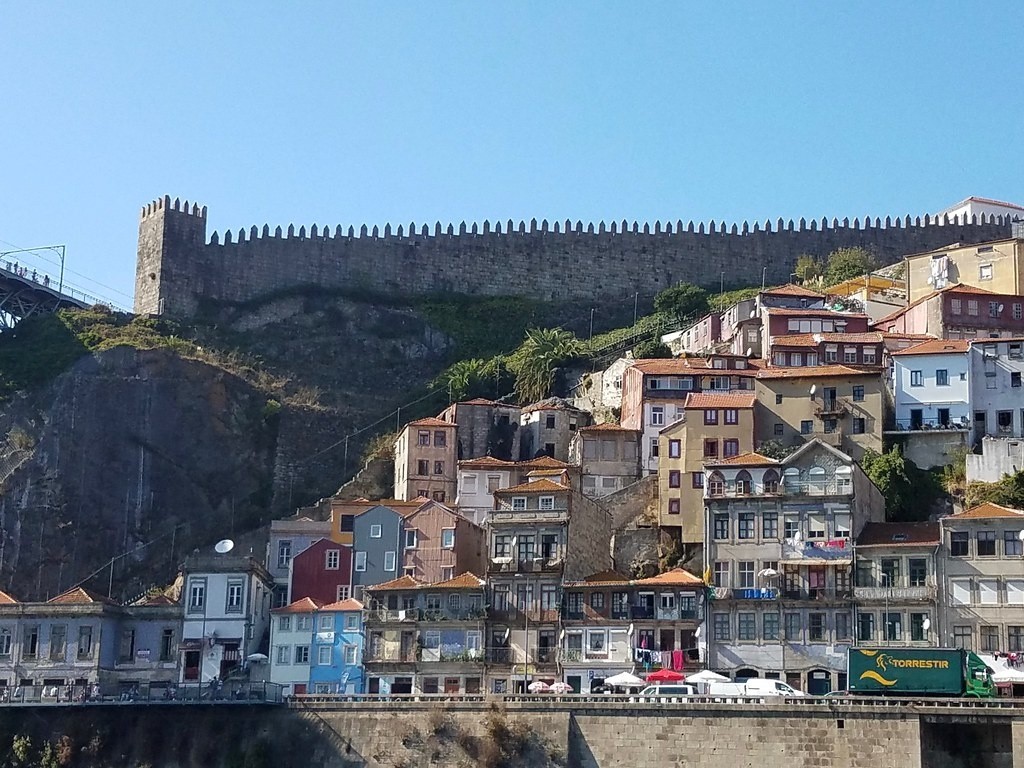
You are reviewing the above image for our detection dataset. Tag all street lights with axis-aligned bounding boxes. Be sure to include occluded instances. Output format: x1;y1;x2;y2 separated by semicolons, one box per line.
514;573;529;694
878;573;890;645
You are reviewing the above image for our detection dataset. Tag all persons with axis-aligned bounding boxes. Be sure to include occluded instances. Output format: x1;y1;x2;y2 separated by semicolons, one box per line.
236;683;247;700
93;682;101;696
42;685;59;698
130;685;139;696
2;685;10;702
14;685;22;698
64;684;71;697
208;676;218;701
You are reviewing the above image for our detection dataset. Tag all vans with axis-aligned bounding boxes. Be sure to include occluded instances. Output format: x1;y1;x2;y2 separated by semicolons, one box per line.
636;685;699;702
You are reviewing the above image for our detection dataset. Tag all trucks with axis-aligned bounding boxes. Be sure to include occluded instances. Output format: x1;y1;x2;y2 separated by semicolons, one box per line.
846;647;998;708
709;678;805;703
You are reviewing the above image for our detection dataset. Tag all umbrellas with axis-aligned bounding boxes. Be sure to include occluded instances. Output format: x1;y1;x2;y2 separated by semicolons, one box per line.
247;653;268;664
528;681;574;702
604;669;731;694
758;569;780;587
991;668;1024;698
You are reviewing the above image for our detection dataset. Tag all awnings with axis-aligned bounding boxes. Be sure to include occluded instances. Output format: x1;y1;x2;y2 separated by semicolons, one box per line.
183;637;242;645
778;560;852;565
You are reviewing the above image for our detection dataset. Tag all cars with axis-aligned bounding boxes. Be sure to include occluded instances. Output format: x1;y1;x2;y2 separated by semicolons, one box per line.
822;690;852;704
